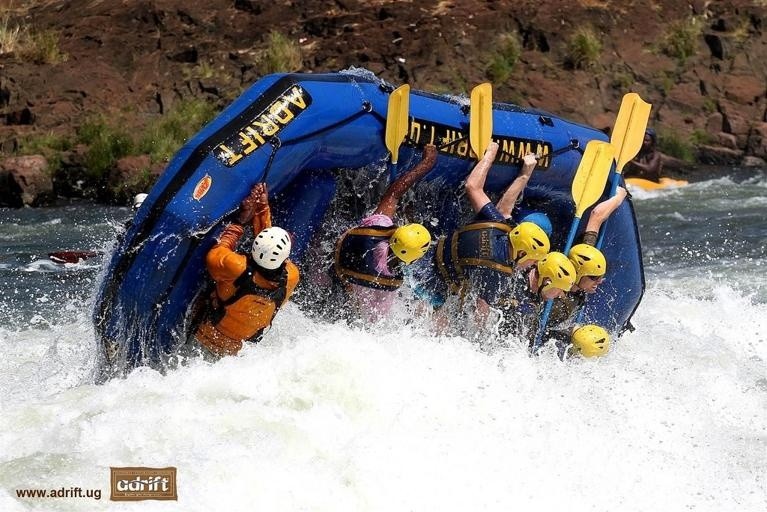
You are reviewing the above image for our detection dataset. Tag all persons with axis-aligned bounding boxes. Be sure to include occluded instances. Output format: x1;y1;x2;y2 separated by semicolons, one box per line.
495;153;578;349
403;140;551;352
305;143;439;329
534;324;609;365
553;183;627;329
188;182;301;366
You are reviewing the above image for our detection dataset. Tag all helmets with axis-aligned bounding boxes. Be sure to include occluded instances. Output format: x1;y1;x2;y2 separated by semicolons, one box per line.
571;325;610;358
568;243;606;287
538;252;577;292
390;223;432;266
509;221;551;263
250;226;293;270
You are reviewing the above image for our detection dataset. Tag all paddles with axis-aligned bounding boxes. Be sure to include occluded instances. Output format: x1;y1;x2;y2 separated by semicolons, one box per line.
532;139;615;353
575;93;652;321
470;82;493;161
385;84;410;183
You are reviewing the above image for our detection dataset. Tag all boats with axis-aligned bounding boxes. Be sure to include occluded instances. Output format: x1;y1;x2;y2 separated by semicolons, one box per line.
89;73;646;384
